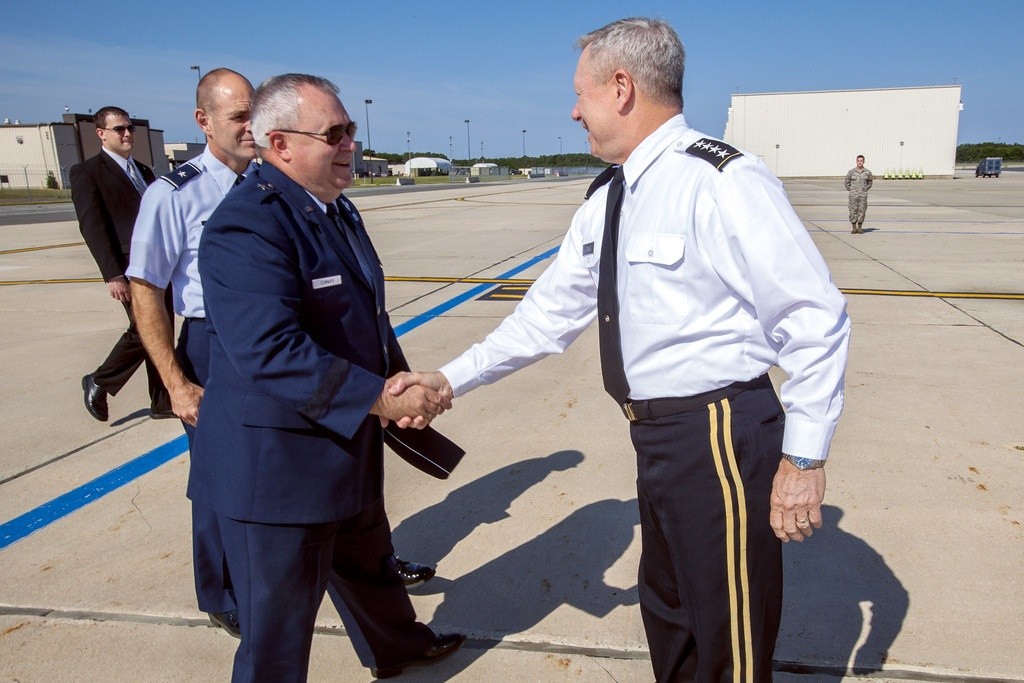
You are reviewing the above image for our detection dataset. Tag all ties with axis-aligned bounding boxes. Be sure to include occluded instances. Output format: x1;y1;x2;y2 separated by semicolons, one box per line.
236;175;246;185
128;161;147;196
597;165;631;407
325;204;353;252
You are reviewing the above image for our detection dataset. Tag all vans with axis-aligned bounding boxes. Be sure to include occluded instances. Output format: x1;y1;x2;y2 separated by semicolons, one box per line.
975;156;1002;178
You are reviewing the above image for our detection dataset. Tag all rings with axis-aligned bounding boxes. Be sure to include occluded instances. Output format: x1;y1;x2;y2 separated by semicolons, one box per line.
796;518;809;523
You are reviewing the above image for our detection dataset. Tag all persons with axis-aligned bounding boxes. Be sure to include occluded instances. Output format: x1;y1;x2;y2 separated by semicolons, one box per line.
186;73;467;683
844;155;873;234
68;106;179;422
125;69;436;638
380;17;850;683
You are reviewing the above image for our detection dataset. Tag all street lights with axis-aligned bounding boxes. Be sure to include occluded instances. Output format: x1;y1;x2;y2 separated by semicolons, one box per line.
558;136;563;158
190;64;210;146
522;130;527;156
448;136;453;168
464;119;471;169
364;99;374;183
406;131;411;160
584;142;588;160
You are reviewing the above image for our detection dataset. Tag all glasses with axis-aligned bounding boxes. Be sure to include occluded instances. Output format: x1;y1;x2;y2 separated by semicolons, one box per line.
265;122;356;144
100;124;137;132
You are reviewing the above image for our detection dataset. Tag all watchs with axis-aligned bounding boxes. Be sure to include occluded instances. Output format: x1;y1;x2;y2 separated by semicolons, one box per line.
783;453;825;470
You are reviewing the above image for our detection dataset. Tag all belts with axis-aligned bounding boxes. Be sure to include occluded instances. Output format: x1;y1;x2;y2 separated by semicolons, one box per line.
623;372;769;422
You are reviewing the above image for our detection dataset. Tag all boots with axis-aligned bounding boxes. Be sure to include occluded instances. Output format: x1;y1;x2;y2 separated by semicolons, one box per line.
857;223;863;233
851;223;857;233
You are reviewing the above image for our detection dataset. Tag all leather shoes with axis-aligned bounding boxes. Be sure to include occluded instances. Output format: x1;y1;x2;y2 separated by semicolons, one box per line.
397;559;432;588
149;410;178;419
371;633;464;678
81;373;109;421
208;610;242;639
